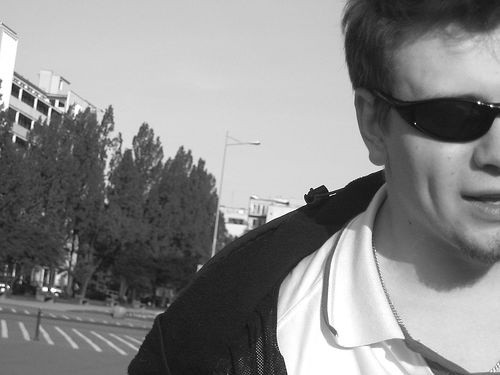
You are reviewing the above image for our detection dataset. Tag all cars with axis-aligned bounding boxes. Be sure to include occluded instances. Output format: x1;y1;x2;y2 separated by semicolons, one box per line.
139;295;170;308
41;285;66;298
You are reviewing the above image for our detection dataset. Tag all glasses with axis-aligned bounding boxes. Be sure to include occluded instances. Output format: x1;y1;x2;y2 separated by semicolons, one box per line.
367;87;500;144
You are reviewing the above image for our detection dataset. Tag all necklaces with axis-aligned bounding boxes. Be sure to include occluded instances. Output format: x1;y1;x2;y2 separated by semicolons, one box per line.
370;238;499;375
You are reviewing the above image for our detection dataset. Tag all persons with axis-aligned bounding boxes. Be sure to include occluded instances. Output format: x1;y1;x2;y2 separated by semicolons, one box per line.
126;0;500;374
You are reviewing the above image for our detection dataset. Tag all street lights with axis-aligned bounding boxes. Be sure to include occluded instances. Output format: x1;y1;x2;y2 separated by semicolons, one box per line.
246;188;289;236
210;128;261;256
